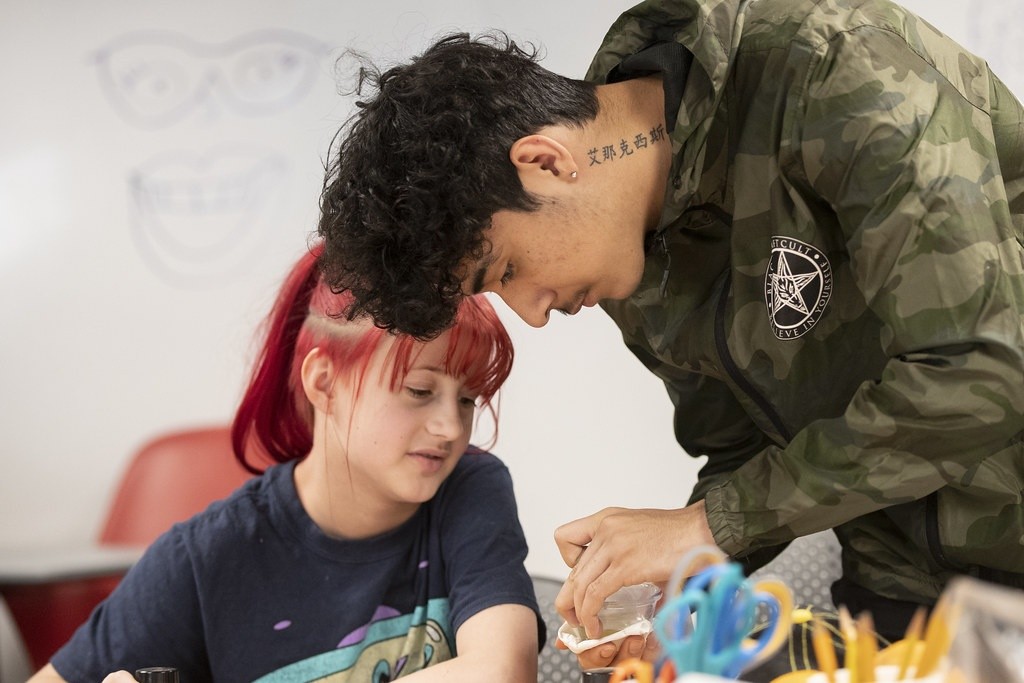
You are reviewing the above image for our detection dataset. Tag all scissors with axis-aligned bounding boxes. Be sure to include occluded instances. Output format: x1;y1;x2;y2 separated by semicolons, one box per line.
608;546;792;683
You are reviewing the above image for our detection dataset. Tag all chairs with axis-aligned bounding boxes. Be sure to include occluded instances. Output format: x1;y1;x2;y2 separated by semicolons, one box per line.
96;417;278;550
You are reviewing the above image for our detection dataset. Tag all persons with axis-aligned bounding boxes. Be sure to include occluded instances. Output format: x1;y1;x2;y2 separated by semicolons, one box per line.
317;0;1023;672
24;242;549;683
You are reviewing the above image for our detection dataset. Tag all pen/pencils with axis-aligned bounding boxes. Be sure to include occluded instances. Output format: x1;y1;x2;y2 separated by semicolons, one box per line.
811;604;929;683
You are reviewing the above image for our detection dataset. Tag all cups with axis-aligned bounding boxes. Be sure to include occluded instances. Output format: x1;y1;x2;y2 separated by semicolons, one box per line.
578;583;663;641
